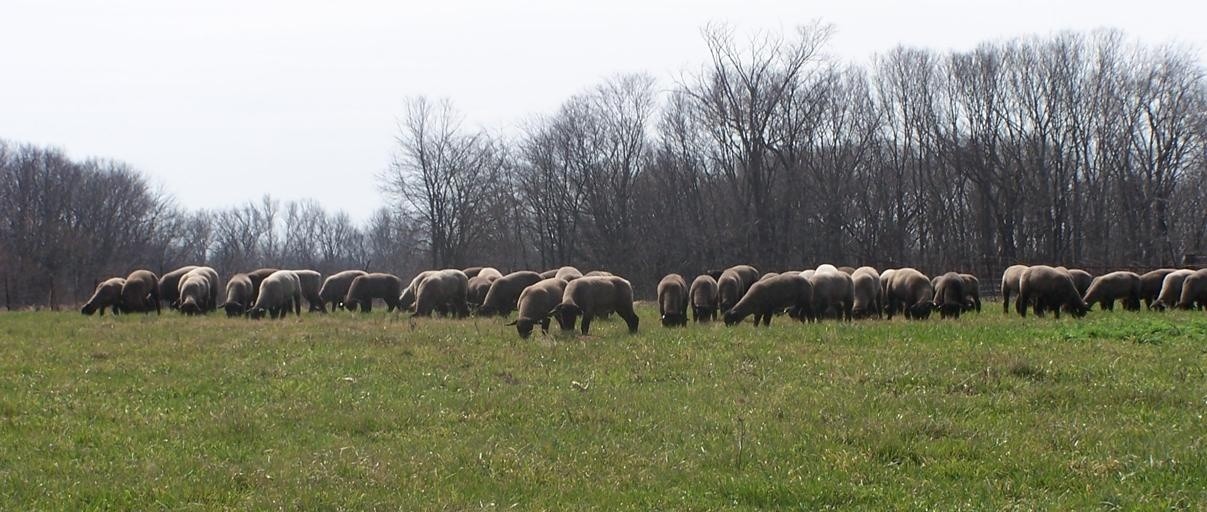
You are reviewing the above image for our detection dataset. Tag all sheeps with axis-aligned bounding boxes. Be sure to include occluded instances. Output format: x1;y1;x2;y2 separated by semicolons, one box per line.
656;265;1201;329
79;265;640;340
